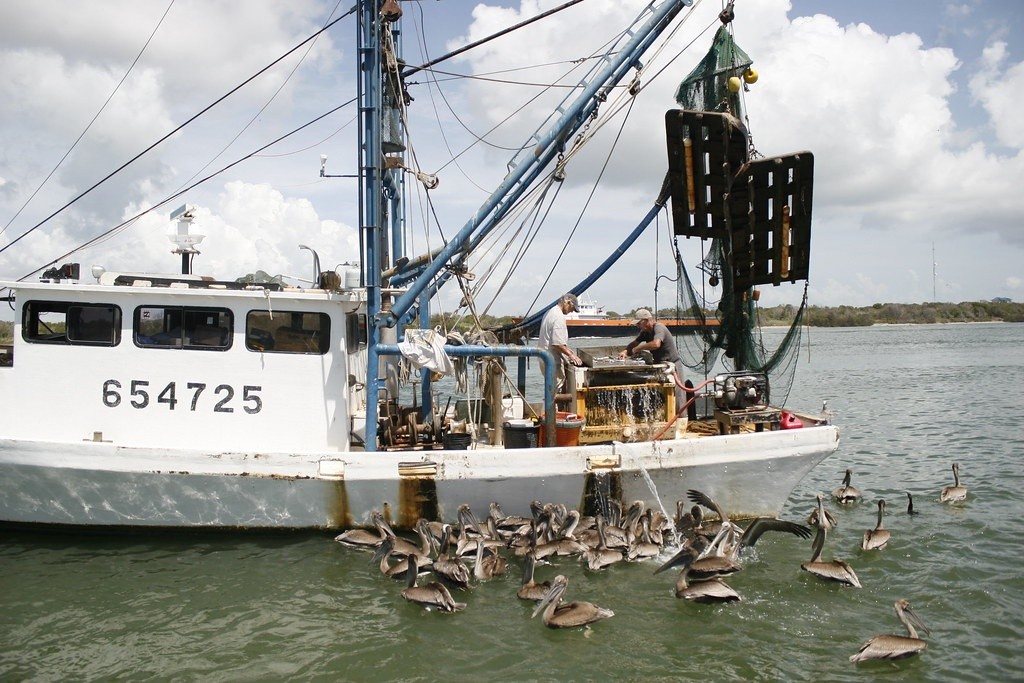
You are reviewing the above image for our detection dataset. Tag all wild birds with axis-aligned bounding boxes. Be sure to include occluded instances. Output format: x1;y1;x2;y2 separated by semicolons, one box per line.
518;550;553;600
849;599;930;661
653;546;741;605
333;487;837;592
860;499;890;551
905;489;919;515
799;524;865;589
401;553;467;614
531;574;613;629
940;463;967;504
836;470;864;507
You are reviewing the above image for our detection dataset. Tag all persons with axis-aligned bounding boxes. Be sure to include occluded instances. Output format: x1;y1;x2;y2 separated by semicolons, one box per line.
537;293;582;413
618;308;686;417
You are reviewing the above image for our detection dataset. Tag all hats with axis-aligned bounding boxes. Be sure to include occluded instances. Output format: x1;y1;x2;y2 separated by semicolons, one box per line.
630;309;653;324
562;294;580;313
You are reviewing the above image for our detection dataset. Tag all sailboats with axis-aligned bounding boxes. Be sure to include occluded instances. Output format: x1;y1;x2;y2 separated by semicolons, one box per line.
0;1;841;531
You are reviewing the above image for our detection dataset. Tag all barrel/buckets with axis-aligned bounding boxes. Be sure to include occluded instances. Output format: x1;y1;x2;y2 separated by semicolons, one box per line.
539;411;587;447
443;433;472;450
503;422;540;449
